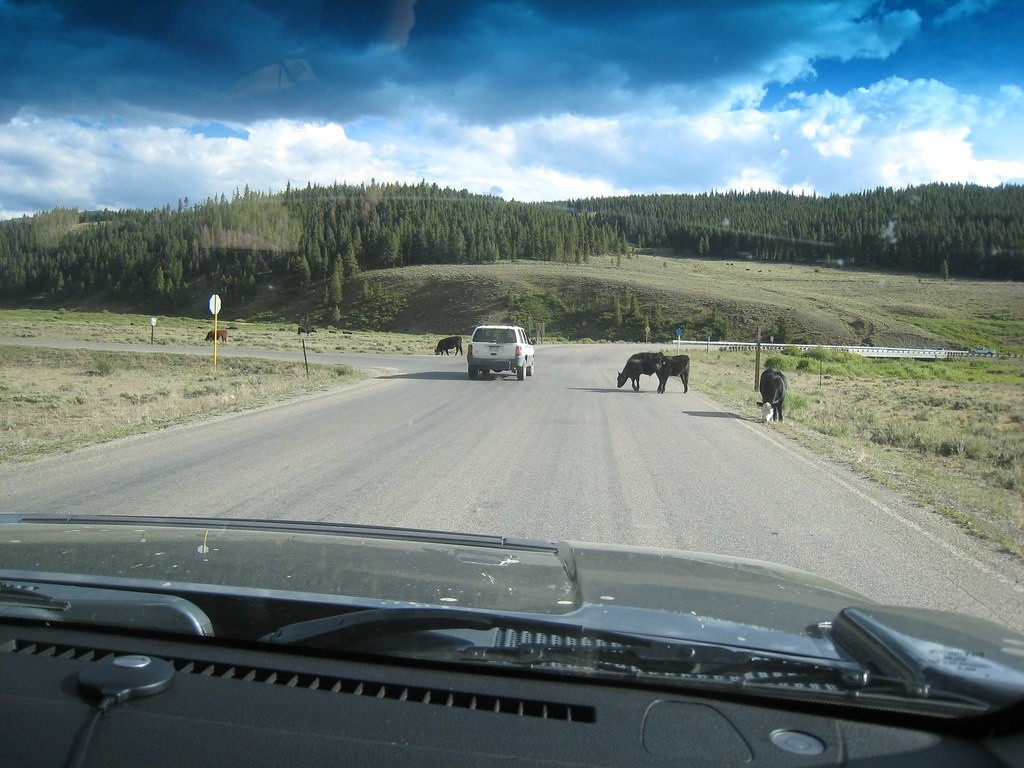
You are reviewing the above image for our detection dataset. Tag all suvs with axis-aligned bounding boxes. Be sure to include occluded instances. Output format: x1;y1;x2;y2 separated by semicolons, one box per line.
467;323;535;380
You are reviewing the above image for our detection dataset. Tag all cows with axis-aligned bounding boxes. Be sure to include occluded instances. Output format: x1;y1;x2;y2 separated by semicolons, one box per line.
434;336;463;356
617;352;690;395
297;325;317;335
756;368;787;423
203;329;228;342
